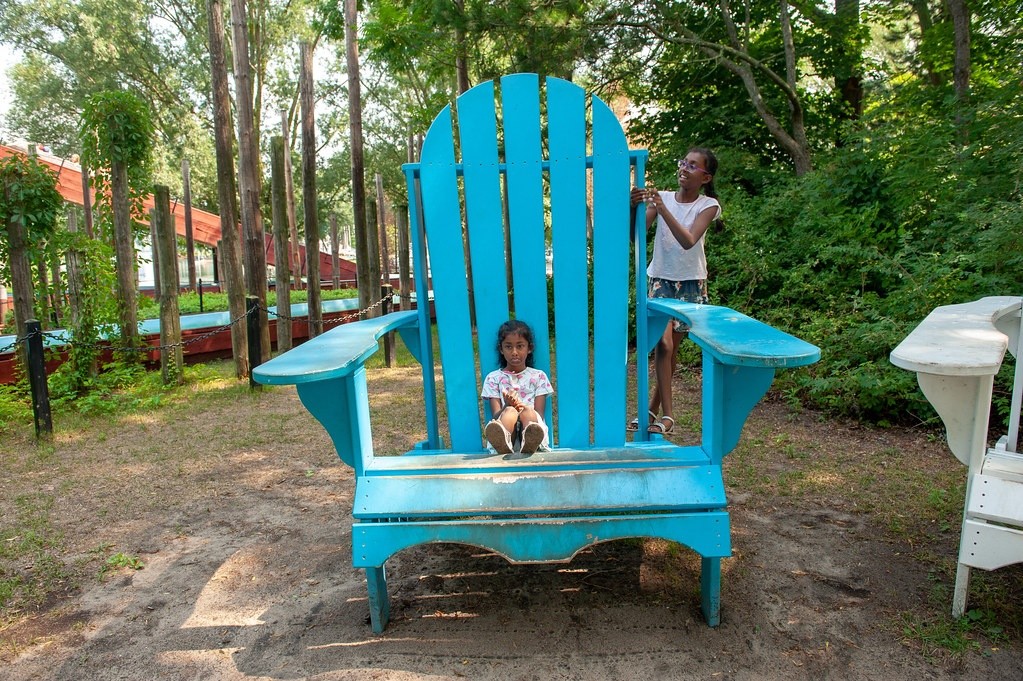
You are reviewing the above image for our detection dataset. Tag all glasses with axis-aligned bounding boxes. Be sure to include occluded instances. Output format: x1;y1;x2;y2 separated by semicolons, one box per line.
677;159;708;173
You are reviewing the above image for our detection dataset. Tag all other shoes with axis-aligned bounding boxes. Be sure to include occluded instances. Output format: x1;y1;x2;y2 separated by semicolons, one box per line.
520;422;544;453
484;421;514;453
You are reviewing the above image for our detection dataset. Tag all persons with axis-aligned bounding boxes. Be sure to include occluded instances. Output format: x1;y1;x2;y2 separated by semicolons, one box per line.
629;144;723;435
479;319;553;454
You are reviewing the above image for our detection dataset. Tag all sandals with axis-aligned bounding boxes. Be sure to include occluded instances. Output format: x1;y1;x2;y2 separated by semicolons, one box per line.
647;415;675;434
626;409;657;431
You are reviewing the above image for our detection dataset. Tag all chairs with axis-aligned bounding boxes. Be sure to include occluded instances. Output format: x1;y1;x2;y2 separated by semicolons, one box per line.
889;295;1023;618
253;73;821;633
